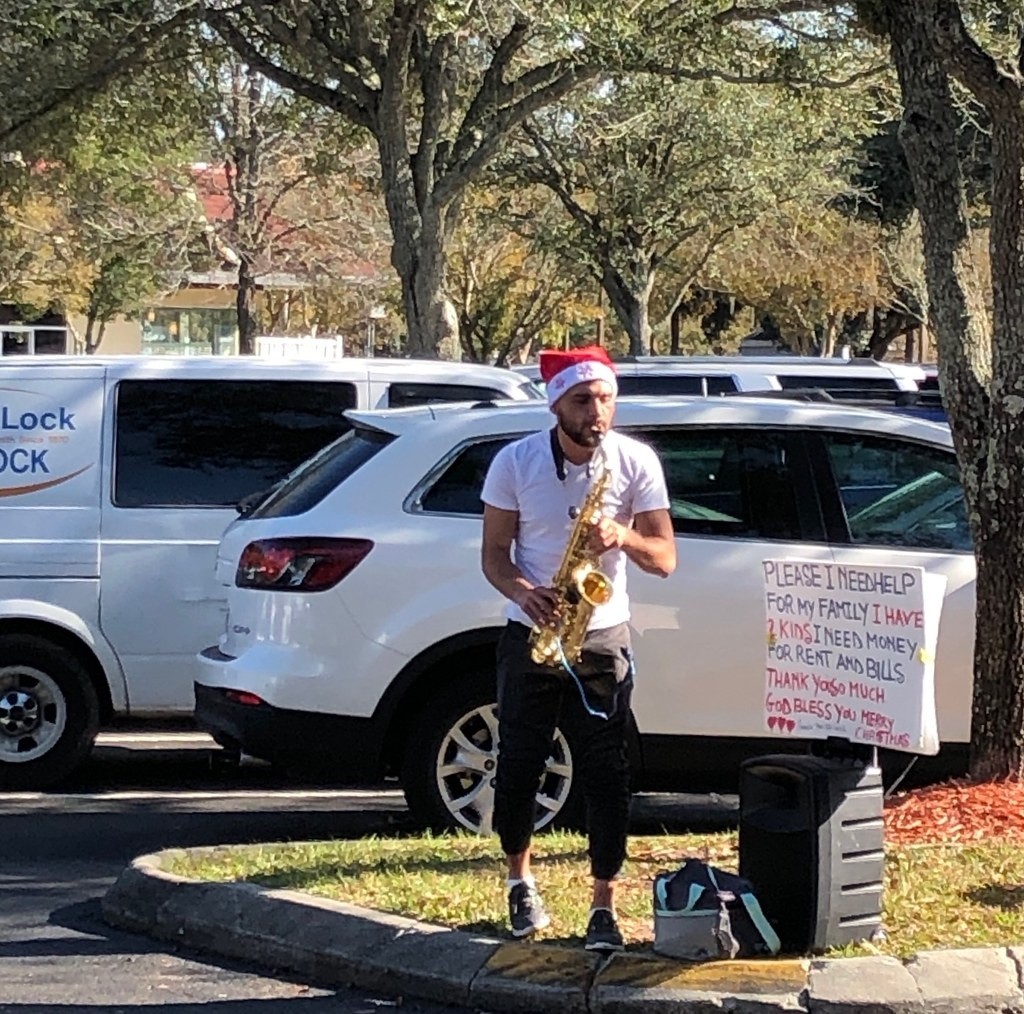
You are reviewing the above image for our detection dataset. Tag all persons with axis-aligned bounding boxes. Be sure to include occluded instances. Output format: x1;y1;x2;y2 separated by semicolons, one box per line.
479;345;678;951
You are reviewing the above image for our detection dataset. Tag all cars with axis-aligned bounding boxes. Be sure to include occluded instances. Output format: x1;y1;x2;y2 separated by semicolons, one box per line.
517;354;953;506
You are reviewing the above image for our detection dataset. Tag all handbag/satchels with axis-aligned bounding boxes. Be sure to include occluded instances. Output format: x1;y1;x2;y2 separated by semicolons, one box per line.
654;858;780;961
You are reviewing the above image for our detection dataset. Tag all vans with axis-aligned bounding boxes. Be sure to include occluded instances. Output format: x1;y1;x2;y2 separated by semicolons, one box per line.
0;354;743;795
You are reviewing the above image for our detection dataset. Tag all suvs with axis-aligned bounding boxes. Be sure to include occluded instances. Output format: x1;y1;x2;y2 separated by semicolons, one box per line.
192;394;974;838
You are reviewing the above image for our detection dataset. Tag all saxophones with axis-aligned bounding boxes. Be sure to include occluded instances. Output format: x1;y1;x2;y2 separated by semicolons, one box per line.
525;424;619;672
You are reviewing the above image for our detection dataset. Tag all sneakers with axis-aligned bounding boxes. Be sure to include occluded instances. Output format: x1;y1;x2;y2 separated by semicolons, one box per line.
508;884;551;936
585;909;625;951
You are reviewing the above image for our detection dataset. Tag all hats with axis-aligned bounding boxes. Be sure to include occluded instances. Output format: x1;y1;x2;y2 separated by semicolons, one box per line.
539;346;618;415
